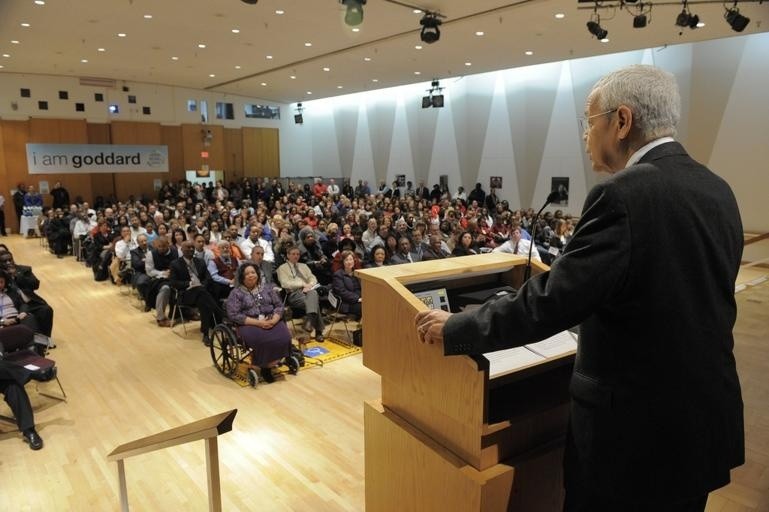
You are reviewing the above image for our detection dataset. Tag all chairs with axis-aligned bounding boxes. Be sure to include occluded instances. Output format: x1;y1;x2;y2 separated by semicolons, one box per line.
169;287;201;338
1;324;67;403
283;282;323;337
324;283;360;345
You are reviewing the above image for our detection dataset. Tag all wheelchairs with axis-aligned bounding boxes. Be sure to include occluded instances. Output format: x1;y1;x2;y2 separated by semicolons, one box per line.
208;298;300;386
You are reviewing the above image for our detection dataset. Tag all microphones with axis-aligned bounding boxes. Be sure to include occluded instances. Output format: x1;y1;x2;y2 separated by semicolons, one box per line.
522;190;559;282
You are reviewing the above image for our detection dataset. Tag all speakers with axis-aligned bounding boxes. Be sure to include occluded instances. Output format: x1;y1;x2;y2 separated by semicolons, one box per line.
440;175;448;193
432;96;444;107
422;96;430;108
295;115;303;123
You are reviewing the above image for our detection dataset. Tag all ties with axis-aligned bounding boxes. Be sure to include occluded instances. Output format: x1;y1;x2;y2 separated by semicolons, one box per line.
514;242;518;254
293;263;304;280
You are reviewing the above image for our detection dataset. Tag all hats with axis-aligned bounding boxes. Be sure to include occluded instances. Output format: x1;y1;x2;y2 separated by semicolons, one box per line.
337;237;356;252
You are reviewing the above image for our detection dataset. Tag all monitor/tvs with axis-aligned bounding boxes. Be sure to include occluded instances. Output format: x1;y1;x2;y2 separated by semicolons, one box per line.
410;286;453;312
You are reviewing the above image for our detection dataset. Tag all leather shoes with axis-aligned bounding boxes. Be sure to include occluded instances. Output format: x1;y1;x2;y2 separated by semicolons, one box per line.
158;319;175;327
315;335;324;342
302;325;313;333
202;336;212;347
30;366;58;382
260;367;273;383
22;429;43;450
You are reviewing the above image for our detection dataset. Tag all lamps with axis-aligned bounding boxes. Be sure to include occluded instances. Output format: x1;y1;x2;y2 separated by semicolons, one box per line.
338;0;369;27
418;7;442;44
575;1;752;40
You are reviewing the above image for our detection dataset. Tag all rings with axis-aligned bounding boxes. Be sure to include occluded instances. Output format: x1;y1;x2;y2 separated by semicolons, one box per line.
419;326;425;334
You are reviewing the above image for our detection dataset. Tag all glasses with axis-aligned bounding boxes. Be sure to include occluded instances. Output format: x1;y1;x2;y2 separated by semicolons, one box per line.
579;109;616;130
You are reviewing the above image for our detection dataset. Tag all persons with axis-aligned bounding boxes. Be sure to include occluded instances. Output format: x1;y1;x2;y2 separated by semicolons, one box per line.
0;174;584;382
0;356;58;449
413;64;746;511
